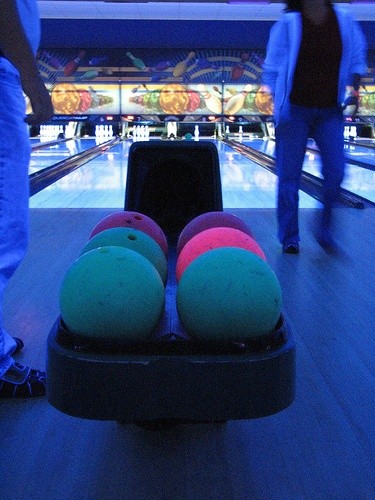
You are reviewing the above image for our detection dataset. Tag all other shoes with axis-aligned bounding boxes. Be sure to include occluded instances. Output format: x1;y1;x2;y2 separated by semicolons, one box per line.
283;241;298;254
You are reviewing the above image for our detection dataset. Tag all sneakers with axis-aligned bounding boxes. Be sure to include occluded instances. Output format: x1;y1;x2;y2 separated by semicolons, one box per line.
0;337;47;399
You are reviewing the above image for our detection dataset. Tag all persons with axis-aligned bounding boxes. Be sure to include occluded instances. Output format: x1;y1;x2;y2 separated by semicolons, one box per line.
261;0;370;255
0;0;55;400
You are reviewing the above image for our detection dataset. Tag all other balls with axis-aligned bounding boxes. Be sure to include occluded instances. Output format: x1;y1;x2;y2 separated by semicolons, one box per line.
185;132;192;139
59;211;282;335
